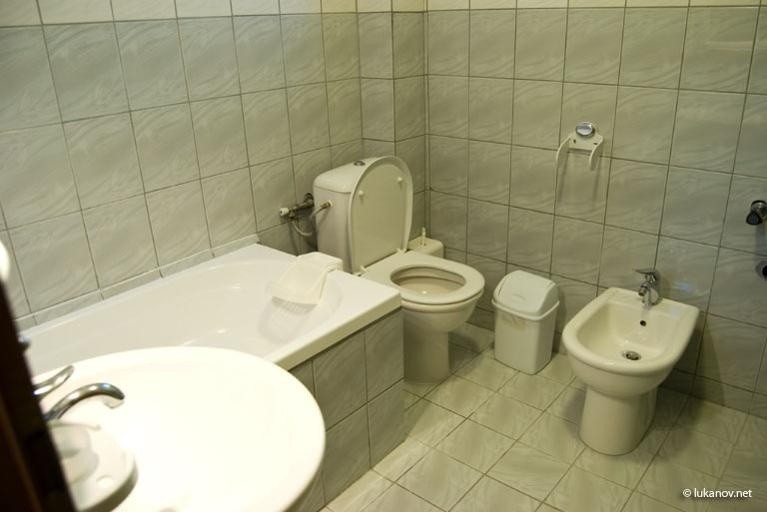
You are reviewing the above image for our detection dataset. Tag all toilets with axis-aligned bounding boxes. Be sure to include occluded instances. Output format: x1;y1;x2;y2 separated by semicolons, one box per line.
313;154;486;383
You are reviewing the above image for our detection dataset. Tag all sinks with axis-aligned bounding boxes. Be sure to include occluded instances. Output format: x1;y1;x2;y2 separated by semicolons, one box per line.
38;345;326;512
562;288;703;390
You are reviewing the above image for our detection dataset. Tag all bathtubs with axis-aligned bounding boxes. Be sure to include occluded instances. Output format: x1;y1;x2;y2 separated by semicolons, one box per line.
14;244;404;504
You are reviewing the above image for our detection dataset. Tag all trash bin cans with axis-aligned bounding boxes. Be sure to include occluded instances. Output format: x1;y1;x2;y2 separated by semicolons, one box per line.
492;269;561;375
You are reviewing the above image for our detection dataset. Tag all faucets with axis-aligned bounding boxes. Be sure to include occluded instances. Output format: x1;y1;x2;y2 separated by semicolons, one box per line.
634;264;665;307
32;365;126;429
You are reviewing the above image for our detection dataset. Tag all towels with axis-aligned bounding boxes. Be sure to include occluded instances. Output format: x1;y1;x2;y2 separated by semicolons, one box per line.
273;252;344;306
